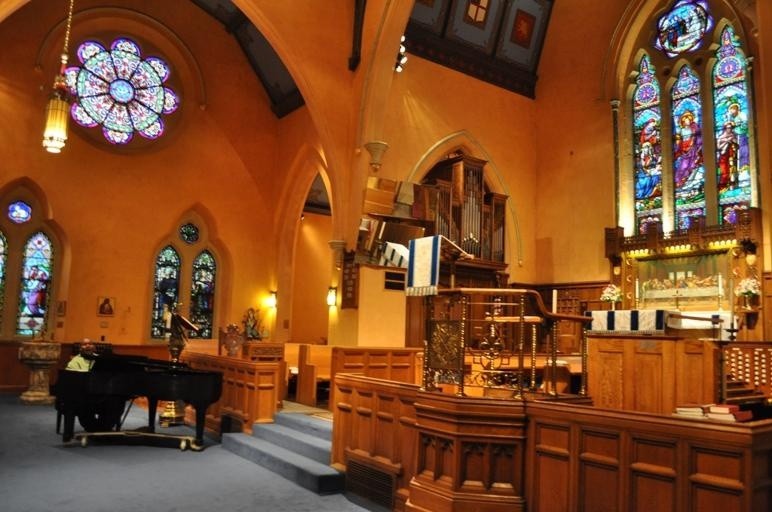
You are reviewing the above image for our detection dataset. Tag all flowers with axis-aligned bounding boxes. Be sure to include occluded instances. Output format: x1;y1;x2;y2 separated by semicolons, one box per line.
733;278;762;299
599;283;623;303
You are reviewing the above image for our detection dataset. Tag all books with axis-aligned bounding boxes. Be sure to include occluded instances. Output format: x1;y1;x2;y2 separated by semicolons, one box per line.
671;400;771;422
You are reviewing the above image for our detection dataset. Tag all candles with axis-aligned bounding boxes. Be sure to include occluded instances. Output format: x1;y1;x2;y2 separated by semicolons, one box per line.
718;272;723;295
635;278;639;299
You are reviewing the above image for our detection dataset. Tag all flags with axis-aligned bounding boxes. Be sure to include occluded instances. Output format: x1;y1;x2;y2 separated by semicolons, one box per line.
404;233;441;297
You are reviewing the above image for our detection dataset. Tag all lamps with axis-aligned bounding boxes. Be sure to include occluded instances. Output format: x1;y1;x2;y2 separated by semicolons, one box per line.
41;0;76;155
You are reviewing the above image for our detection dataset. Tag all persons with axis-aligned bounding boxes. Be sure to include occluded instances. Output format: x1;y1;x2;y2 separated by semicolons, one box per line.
635;103;751;199
64;336;117;434
244;308;261;339
99;297;114;315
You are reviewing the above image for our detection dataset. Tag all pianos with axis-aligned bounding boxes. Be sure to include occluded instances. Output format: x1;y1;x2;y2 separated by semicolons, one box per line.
54;354;223;452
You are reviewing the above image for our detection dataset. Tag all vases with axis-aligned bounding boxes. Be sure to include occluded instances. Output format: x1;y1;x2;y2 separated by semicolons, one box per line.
611;301;615;310
743;295;752;311
